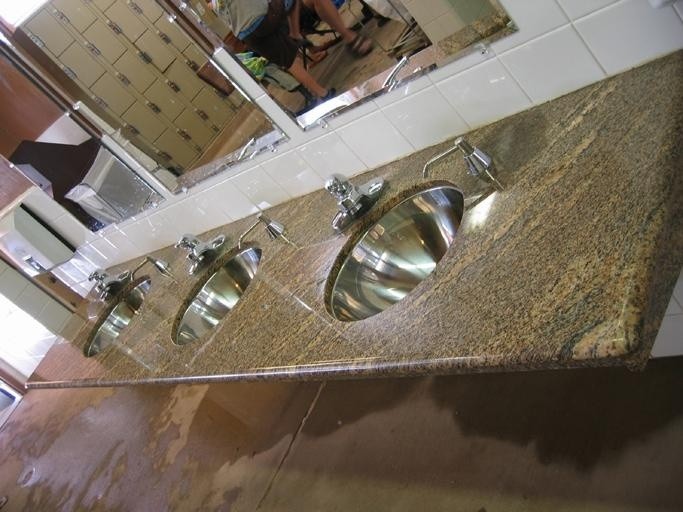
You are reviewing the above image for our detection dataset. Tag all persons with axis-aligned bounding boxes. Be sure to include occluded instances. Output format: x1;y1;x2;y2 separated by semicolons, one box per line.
204;0;391;109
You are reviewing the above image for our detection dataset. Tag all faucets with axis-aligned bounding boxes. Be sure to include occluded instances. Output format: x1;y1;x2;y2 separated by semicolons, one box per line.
236;212;285;251
236;137;258;160
421;137;493;180
131;255;169;282
381;57;410;90
142;190;156;209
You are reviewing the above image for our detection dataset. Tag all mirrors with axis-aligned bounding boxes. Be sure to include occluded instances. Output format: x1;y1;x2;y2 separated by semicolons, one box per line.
182;0;520;132
0;0;290;196
0;48;165;234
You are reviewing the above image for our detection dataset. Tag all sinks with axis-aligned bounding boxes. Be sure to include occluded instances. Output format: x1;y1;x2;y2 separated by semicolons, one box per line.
169;240;262;347
323;178;464;320
82;274;152;357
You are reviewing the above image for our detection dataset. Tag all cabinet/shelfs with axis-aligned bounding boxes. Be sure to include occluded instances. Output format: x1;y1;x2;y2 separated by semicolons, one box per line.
13;0;248;173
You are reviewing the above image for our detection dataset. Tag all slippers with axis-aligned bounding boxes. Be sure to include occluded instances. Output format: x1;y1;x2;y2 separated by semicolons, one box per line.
346;31;373;58
315;88;336;106
